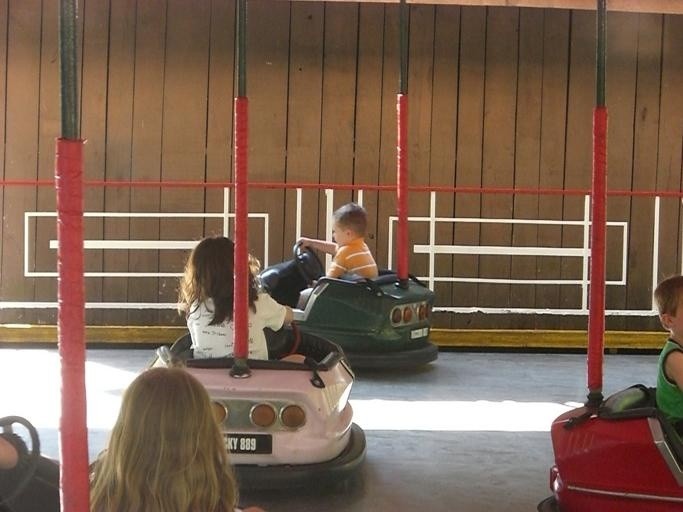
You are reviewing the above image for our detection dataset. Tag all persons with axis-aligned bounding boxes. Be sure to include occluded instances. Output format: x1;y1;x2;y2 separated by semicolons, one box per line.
175;236;294;360
297;202;379;310
0;436;19;471
87;367;241;511
653;274;683;419
